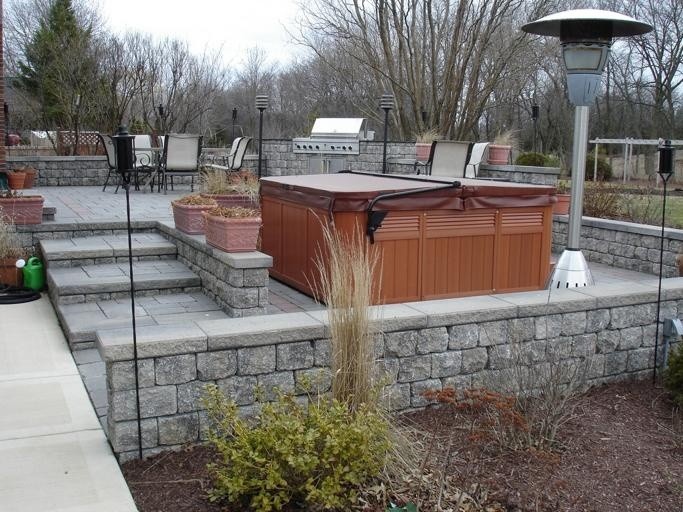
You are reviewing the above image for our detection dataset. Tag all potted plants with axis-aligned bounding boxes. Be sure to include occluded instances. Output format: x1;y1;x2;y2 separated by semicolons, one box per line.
552;181;572;214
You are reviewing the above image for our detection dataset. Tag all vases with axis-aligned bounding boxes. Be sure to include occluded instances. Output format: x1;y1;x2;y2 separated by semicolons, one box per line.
415;143;432;160
488;145;511;165
0;169;45;286
171;191;262;252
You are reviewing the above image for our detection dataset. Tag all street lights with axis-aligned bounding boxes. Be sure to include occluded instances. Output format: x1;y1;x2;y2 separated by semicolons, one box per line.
521;9;653;289
111;123;142;457
651;137;675;393
157;104;165;135
380;94;395;173
254;94;270;182
531;102;540;165
232;107;238;142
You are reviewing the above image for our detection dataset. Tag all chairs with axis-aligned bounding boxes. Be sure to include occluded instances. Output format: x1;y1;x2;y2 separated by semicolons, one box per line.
97;129;253;194
